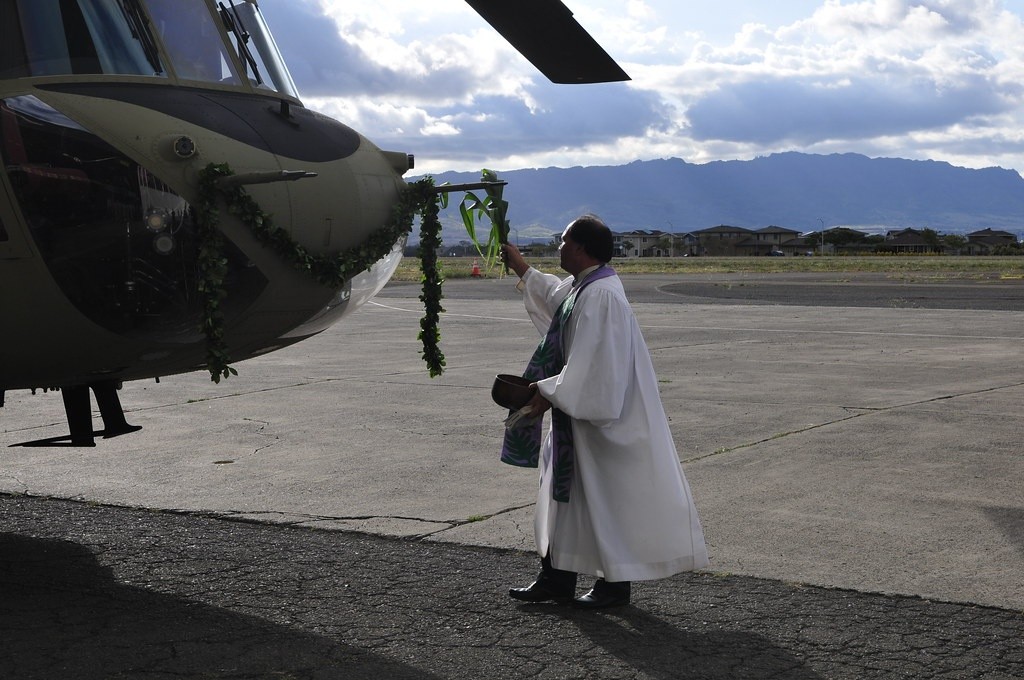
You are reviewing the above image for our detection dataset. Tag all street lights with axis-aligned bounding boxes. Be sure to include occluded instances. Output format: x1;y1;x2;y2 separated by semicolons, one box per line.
512;227;519;248
664;220;675;258
816;217;824;259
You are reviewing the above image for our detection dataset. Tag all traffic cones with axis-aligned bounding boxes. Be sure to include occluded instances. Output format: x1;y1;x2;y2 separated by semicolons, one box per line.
470;255;482;278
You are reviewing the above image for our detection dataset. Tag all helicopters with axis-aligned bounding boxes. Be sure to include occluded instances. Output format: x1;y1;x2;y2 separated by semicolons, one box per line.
2;0;634;447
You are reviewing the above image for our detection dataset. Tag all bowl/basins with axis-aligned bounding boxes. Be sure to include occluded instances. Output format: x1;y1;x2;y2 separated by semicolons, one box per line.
492;374;536;410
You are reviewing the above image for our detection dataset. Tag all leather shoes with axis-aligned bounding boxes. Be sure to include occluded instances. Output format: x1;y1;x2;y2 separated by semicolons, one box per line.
510;572;574;603
575;589;630;608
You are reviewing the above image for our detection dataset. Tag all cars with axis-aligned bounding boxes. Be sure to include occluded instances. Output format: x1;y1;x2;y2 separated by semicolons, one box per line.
803;251;815;257
766;250;785;256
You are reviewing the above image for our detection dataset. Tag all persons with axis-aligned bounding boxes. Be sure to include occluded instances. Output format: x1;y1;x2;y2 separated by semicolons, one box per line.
497;211;653;613
